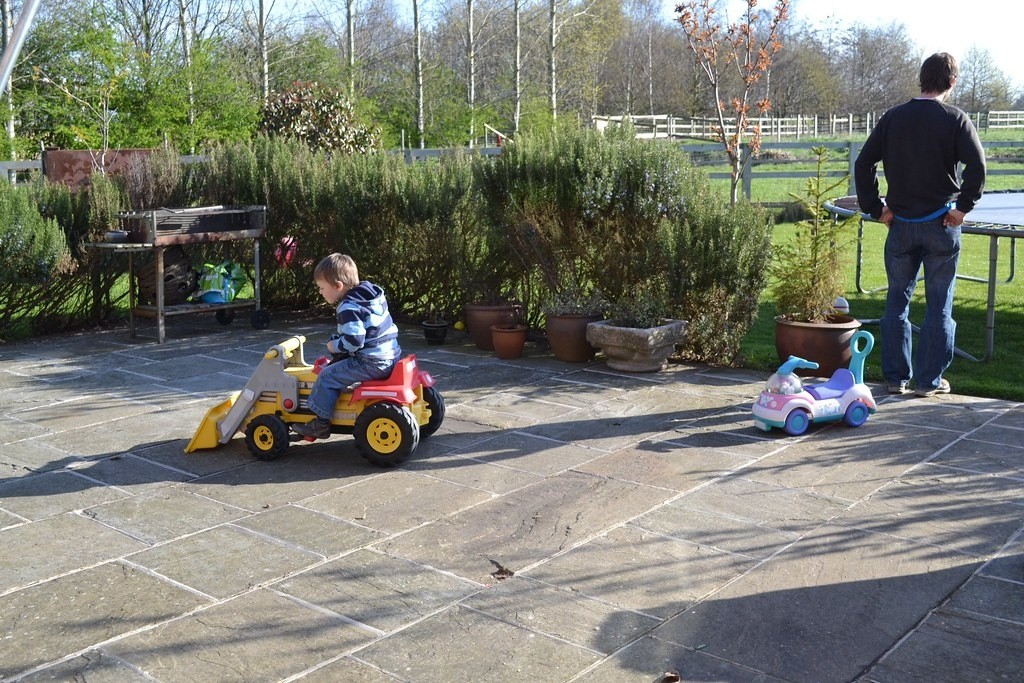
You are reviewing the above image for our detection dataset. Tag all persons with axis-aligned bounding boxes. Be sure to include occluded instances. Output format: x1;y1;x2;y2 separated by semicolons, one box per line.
854;53;986;397
292;253;402;439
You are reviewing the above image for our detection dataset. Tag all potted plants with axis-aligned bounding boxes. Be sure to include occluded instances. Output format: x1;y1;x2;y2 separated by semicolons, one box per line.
766;144;866;379
422;311;449;345
488;305;528;359
540;278;610;362
584;280;690;373
463;268;521;352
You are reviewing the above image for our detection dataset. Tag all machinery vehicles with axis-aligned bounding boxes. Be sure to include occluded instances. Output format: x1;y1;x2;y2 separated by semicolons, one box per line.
182;330;443;466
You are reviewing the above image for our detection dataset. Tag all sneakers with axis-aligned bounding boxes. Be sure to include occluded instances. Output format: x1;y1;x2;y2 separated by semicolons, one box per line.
923;378;951;402
292;418;331;439
889;381;904;393
